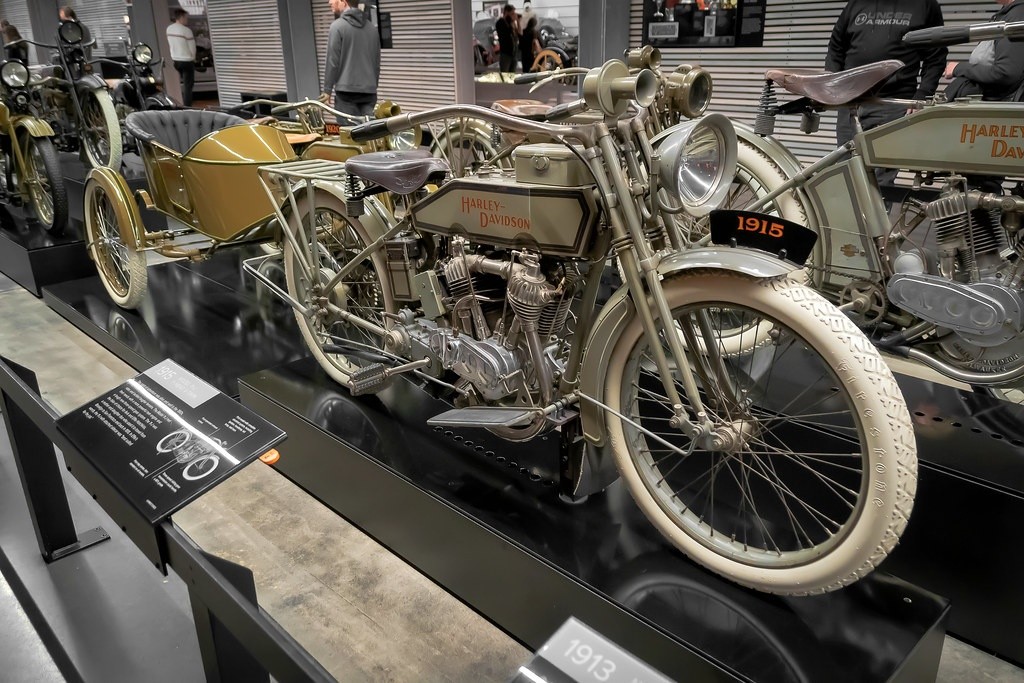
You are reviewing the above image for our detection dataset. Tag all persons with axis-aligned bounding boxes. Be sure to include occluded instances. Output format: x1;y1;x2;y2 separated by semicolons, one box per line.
315;0;382;127
823;0;948;213
165;9;197;108
495;0;539;72
57;6;93;71
0;19;29;65
941;0;1024;101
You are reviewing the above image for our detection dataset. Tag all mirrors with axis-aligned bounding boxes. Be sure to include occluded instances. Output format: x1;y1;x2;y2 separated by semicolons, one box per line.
471;0;580;107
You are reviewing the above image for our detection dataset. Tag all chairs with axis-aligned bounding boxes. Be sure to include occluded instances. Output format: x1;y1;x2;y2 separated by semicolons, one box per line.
125;109;247;155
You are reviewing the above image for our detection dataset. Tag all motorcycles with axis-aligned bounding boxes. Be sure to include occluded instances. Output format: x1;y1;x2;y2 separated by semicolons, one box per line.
0;19;179;234
240;19;1024;599
